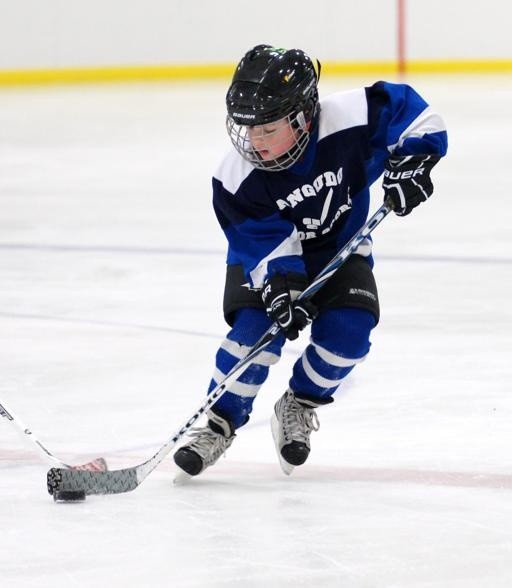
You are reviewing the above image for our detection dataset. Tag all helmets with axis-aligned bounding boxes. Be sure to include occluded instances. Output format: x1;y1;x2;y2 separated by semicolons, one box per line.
224;43;321;172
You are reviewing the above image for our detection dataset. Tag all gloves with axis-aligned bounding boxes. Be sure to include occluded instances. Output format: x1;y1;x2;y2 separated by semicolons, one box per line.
261;273;320;341
383;156;435;216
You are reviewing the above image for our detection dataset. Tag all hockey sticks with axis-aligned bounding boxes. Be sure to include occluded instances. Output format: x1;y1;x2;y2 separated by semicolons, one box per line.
1;403;108;473
48;202;391;493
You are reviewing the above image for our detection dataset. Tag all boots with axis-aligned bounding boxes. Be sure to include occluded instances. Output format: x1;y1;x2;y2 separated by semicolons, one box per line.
173;407;236;475
268;387;320;465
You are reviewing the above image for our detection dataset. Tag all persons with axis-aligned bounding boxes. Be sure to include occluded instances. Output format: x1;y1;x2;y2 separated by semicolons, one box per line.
172;44;448;476
